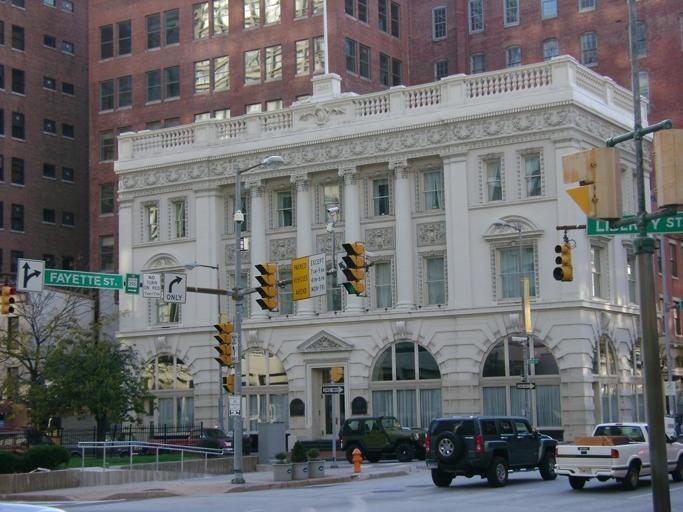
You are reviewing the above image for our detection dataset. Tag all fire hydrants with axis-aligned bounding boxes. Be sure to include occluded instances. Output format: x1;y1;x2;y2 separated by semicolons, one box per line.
351;448;362;472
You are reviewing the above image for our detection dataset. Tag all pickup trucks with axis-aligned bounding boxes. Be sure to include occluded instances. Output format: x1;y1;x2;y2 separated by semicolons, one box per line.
551;421;682;488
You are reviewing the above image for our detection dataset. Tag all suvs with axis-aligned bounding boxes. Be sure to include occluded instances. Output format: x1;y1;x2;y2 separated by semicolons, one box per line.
426;414;556;487
338;415;431;463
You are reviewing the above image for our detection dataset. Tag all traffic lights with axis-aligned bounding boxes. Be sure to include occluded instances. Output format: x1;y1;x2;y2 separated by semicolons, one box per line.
341;242;364;295
211;322;232;369
254;263;276;312
223;375;235;395
561;147;624;220
552;241;572;282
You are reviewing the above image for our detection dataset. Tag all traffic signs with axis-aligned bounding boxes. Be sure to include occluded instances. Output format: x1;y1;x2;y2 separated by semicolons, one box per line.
322;385;345;394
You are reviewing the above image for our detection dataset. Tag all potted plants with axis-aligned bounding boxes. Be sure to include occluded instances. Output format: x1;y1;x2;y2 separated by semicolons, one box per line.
271;440;326;481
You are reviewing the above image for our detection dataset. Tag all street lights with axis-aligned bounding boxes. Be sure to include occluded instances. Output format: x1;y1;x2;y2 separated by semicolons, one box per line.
488;217;537;432
230;156;288;485
